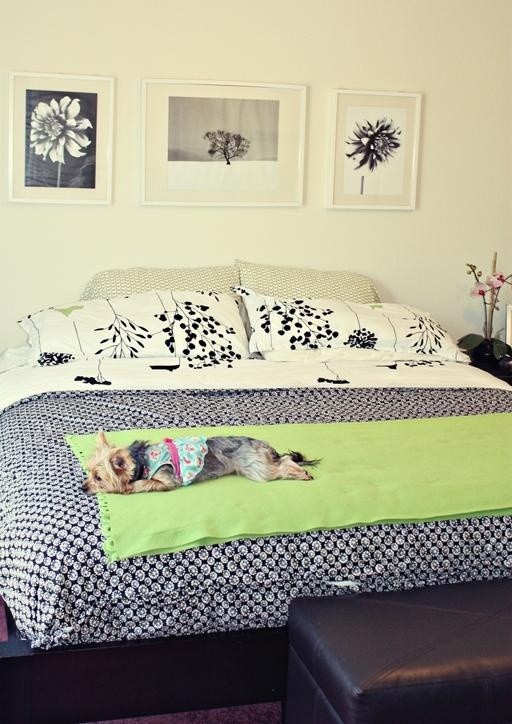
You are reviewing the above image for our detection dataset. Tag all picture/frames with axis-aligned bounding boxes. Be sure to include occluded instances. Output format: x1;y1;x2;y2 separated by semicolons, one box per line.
8;70;424;214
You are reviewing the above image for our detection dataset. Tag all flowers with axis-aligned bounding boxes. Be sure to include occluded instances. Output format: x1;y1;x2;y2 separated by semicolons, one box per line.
456;251;510;366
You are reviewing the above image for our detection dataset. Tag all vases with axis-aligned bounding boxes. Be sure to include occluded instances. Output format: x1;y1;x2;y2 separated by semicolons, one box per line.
474;349;507;377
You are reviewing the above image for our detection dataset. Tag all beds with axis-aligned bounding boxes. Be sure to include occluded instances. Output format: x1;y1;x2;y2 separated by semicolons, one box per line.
0;258;512;724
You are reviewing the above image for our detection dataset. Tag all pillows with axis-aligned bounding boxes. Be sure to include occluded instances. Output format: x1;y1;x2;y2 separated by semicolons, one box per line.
232;286;471;364
236;262;381;304
16;291;254;370
81;263;240;299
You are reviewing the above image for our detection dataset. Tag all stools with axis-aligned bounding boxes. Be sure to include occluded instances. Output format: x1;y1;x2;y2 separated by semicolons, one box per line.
279;578;511;724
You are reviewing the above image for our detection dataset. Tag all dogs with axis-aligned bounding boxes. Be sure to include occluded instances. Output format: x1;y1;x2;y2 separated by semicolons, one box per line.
80;428;324;495
